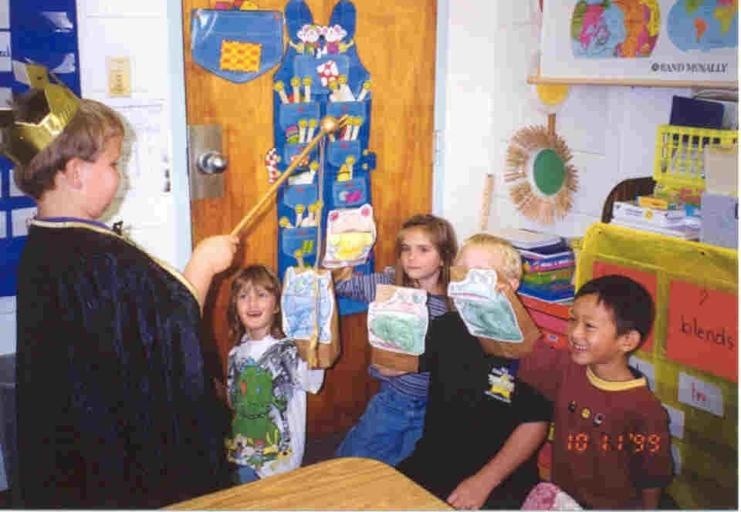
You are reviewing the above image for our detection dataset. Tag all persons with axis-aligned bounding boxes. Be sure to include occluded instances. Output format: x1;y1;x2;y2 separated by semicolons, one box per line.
329;214;460;469
220;261;326;491
374;234;556;509
512;273;680;510
5;89;245;508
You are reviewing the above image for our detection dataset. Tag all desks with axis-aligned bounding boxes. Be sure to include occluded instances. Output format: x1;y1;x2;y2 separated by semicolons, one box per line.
155;457;456;511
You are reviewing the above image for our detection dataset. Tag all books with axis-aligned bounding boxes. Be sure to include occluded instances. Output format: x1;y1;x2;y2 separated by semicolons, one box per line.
611;201;703;243
